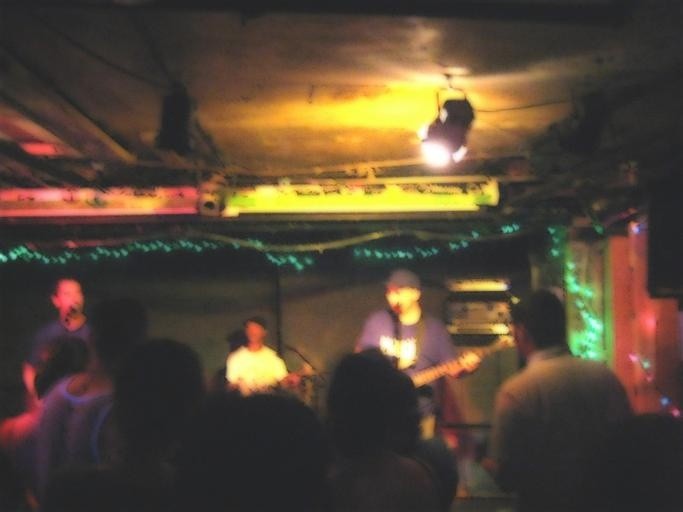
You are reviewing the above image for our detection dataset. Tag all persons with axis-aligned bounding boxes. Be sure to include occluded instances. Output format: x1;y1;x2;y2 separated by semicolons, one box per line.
580;408;682;511
24;274;104;406
0;296;150;511
492;287;631;511
460;337;570;510
224;316;290;395
352;271;454;379
103;338;462;510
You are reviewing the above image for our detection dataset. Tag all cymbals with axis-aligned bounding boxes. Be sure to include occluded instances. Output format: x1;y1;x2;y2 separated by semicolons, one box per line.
294;370;331;376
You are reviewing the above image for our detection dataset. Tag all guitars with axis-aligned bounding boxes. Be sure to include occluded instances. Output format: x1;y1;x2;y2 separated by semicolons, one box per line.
411;333;518;397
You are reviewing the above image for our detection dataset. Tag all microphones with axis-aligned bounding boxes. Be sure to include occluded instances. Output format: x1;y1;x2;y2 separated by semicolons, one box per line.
395;304;403;337
66;303;80;322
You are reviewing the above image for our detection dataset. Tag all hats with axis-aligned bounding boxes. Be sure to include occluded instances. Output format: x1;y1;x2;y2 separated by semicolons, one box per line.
385;269;422;289
246;315;267;327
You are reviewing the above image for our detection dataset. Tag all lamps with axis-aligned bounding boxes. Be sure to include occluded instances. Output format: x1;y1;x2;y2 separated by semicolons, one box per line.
421;99;475;164
